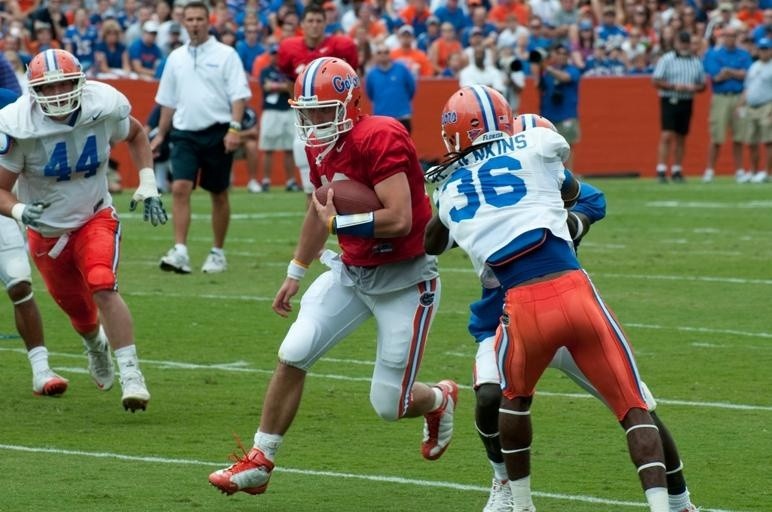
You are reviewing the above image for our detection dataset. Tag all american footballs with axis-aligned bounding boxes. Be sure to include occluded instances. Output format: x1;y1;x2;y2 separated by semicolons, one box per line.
314;179;384;215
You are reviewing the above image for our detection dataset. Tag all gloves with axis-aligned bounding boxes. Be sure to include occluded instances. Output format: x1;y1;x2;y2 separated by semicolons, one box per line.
128;167;169;226
11;200;53;227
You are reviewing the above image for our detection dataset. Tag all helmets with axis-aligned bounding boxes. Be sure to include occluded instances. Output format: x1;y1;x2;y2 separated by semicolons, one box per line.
512;111;558;136
25;49;86;119
287;56;364;150
439;83;514;154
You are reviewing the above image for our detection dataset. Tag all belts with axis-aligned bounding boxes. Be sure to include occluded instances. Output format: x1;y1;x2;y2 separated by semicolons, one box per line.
723;90;741;95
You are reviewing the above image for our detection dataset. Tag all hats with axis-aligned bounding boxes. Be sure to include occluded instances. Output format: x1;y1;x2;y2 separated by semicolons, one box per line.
322;1;483;38
143;20;159;34
675;30;689;42
756;37;772;50
169;22;181;35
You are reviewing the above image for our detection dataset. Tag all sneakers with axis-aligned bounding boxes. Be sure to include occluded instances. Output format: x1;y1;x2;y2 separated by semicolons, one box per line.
420;379;459;461
159;248;192;274
80;324;115;392
201;250;228;273
32;367;69;396
263;182;269;192
481;475;515;512
118;369;151;414
656;166;770;184
247;177;263;193
207;433;276;496
285;182;302;192
